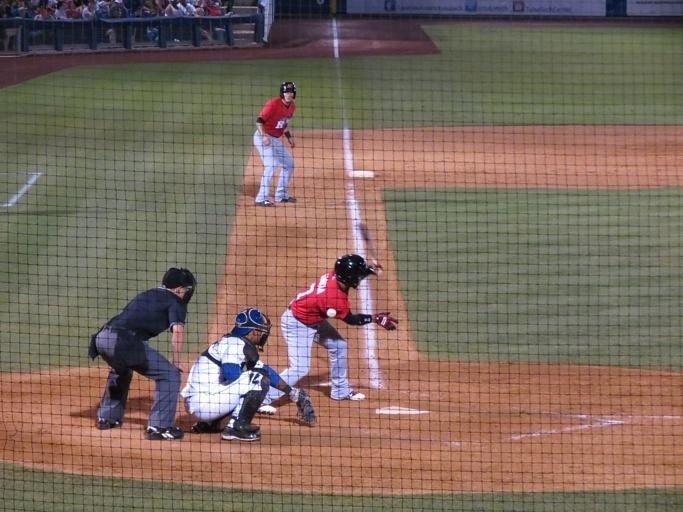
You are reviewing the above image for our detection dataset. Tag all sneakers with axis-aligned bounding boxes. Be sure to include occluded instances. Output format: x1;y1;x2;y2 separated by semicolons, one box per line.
257;403;276;415
97;418;122;430
147;426;184;440
256;200;273;207
222;427;260;441
344;390;365;400
191;420;218;433
283;197;296;202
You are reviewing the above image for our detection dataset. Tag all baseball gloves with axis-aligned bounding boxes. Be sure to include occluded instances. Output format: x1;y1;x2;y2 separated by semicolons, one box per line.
289;387;315;424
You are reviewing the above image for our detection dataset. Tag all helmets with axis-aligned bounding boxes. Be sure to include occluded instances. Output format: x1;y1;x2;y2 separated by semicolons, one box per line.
231;307;272;352
279;81;296;100
334;254;367;288
162;267;196;303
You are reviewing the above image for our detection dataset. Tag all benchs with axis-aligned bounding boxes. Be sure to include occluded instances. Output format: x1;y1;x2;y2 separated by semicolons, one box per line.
212;5;259;38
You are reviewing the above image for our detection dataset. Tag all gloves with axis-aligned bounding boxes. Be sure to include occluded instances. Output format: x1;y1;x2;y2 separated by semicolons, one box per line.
374;311;399;330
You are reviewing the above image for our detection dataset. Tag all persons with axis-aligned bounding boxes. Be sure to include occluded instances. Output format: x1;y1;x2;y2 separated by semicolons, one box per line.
0;0;275;51
179;306;316;443
87;266;196;441
255;253;399;415
251;81;297;207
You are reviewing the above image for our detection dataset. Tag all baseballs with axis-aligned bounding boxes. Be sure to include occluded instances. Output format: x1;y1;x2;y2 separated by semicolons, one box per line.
328;308;337;317
